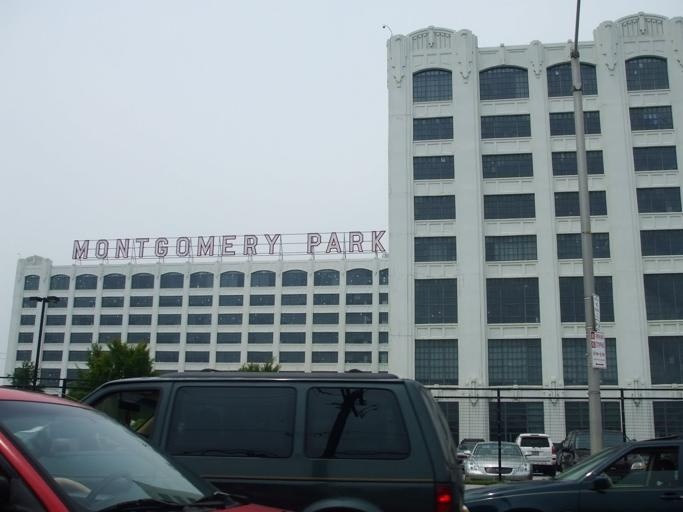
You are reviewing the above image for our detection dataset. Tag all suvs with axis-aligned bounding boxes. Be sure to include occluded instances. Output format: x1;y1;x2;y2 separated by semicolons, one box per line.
75;366;469;512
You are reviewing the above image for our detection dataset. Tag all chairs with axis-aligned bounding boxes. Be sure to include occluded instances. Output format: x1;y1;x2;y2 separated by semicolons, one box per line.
48;477;93;505
192;407;224;455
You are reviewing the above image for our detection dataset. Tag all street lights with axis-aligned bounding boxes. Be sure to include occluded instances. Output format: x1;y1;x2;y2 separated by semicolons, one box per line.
28;295;61;388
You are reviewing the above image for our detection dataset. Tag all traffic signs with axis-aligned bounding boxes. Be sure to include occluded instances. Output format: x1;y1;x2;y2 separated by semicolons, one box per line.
589;292;601;328
588;329;607;369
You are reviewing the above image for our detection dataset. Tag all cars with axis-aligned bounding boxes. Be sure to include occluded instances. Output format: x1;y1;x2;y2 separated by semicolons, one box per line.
456;424;682;512
0;384;289;512
